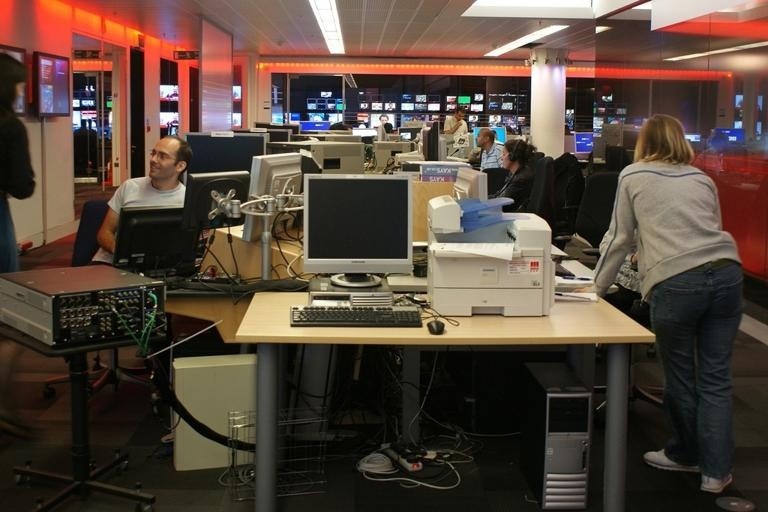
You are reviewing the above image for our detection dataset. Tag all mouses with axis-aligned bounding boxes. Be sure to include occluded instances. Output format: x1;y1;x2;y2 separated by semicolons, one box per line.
428;321;448;336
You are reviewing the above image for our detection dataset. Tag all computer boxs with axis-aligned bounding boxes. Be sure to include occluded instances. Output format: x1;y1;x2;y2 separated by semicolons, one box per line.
308;275;394;310
526;359;592;509
171;353;257;470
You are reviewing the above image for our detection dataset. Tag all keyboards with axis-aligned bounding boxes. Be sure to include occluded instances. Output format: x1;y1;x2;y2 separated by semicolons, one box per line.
290;305;420;327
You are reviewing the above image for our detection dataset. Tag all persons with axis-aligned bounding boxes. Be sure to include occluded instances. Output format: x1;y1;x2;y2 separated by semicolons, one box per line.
443;107;470;137
384;122;394;135
330;123;353;132
0;48;36;277
487;139;538;213
709;129;734;151
373;114;389;129
592;114;746;494
90;135;195;265
469;128;505;171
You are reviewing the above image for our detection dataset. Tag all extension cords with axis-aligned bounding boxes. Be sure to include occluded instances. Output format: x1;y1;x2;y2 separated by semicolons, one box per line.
380;445;426;472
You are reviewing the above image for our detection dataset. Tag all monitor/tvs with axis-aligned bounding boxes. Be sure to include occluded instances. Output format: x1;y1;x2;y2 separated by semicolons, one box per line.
684;132;703;145
185;130;266;177
620;128;640;151
394;142;426;171
303;173;414;288
396;127;423;142
0;42;25;117
112;205;202;279
734;94;763;113
368;112;397;130
473;126;507;152
452;166;488;202
400;92;531;136
423;121;441;161
574;131;593;162
30;48;72;118
75;72;768;288
565;88;628;137
241;152;303;243
74;84;383;172
714;128;745;150
733;120;762;141
184;169;250;229
384;102;397;112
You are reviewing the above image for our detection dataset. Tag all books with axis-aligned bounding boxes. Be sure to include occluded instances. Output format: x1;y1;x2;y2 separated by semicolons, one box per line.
552;255;621;304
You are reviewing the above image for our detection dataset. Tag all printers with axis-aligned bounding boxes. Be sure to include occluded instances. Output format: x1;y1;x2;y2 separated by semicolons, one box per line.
426;193;557;316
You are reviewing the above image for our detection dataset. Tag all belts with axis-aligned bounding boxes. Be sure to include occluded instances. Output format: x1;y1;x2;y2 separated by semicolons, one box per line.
689;258;738;273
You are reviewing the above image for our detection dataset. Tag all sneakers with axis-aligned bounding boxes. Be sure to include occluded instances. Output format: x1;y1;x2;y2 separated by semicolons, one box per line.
0;414;45;440
641;449;703;472
699;471;733;493
